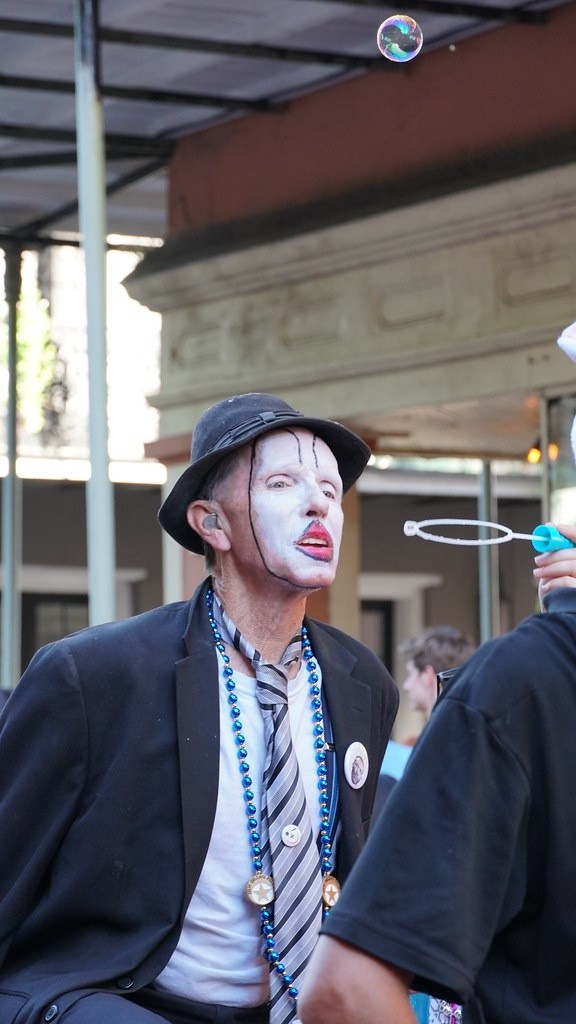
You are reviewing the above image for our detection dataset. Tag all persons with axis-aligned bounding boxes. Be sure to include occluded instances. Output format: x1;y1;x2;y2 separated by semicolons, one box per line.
296;520;576;1024
0;393;400;1024
398;625;474;724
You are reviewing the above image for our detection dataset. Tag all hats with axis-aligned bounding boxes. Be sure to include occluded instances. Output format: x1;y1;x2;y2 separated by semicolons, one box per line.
157;393;371;556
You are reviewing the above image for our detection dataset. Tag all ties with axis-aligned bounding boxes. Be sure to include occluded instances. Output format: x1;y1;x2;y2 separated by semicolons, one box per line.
210;594;321;1024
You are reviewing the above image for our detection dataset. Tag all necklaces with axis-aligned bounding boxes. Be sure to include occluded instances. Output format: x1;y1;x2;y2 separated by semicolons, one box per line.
205;591;333;1010
